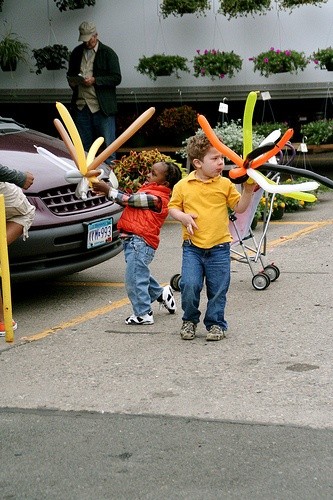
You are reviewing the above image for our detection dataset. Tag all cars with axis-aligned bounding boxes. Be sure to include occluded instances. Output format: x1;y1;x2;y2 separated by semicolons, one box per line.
0;115;127;284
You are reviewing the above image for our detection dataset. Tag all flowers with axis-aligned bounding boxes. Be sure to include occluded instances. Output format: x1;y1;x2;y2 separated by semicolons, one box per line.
108;47;333;223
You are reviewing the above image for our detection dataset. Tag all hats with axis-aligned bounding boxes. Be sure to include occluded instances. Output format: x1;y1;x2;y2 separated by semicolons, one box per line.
78;22;97;41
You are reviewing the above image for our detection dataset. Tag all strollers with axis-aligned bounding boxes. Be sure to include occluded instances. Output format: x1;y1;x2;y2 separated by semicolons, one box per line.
169;140;296;292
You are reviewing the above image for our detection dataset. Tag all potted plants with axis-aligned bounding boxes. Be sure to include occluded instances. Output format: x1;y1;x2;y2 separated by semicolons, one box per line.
0;23;71;75
54;0;96;12
160;0;211;19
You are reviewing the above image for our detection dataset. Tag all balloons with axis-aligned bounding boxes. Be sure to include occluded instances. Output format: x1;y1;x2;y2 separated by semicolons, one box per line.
197;91;333;202
33;101;155;200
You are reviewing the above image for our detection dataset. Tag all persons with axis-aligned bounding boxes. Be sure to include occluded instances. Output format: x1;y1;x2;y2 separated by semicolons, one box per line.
67;21;122;164
167;129;256;342
0;164;36;337
91;161;182;325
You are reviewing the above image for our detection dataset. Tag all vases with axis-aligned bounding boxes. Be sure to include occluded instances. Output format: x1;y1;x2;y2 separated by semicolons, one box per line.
261;206;283;220
152;67;172;76
326;62;333;71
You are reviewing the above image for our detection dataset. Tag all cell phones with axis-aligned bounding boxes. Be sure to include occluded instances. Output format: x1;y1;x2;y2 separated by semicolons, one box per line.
75;74;85;81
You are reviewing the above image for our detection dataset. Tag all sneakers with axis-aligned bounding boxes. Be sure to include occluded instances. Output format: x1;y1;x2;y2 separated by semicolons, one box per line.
125;310;155;325
180;321;196;339
206;325;224;340
159;285;176;314
0;319;18;336
